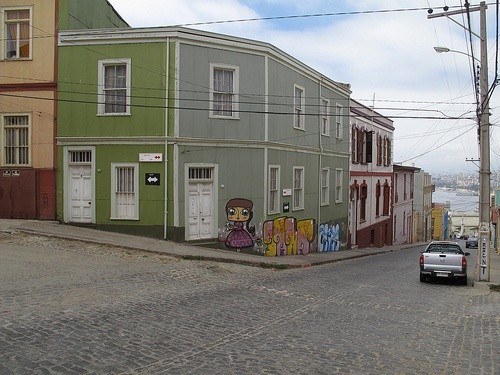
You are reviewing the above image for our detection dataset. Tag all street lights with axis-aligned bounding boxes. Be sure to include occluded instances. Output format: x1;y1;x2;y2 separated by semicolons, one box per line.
433;46;490;283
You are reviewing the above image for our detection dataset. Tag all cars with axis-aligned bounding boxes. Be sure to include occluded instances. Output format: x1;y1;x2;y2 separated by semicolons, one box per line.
466;237;478;248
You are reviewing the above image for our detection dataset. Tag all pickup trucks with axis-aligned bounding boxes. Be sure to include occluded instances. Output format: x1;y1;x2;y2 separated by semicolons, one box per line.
419;240;471;286
